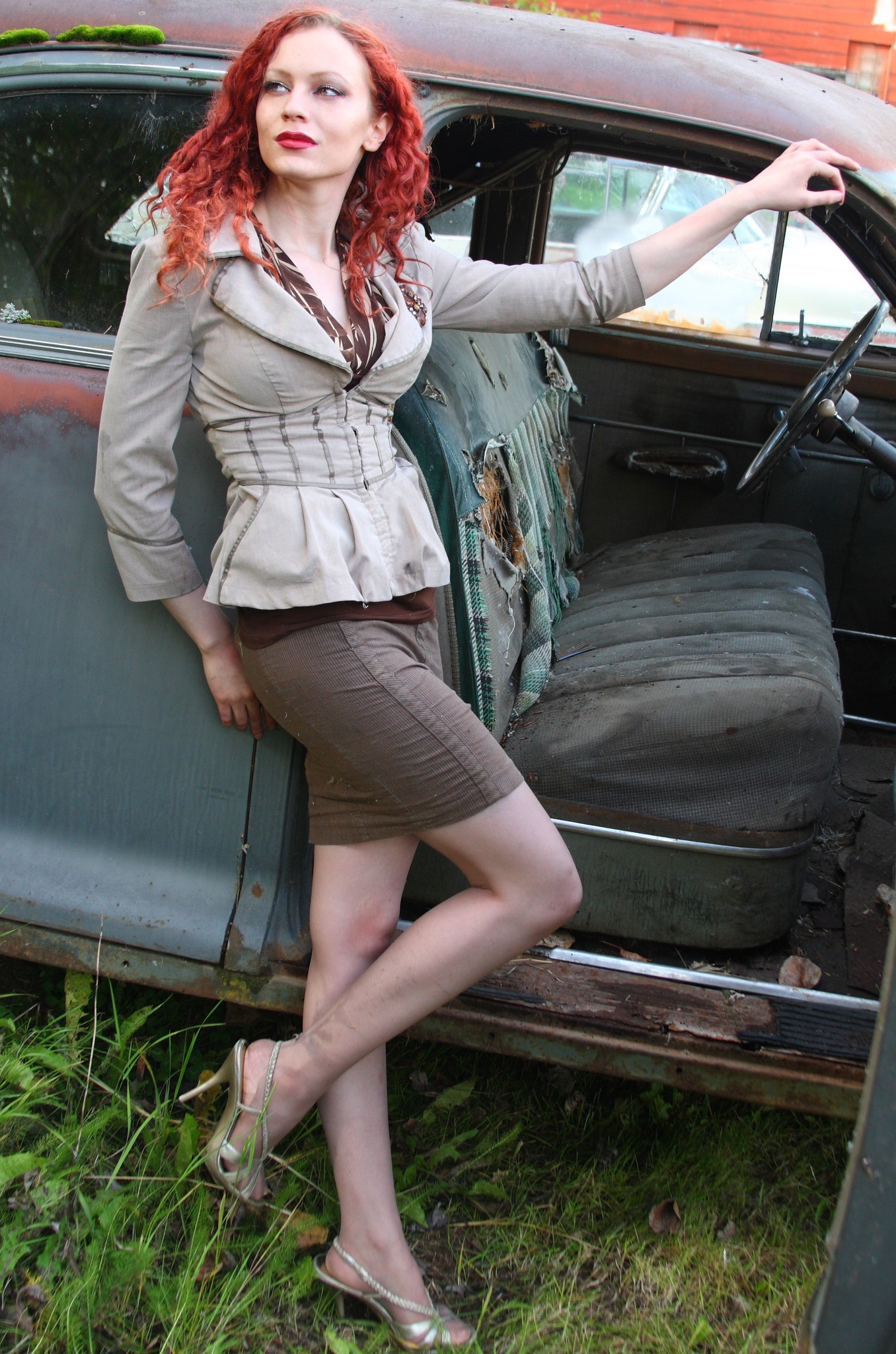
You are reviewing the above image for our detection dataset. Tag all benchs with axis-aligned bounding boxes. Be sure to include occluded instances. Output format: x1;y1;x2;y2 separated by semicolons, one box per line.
388;327;846;946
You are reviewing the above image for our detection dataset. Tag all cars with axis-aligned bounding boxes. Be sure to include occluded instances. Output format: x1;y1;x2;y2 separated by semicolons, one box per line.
1;7;896;1123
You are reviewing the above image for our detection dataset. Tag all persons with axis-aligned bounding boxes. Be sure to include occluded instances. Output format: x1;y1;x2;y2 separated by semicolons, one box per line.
91;9;861;1351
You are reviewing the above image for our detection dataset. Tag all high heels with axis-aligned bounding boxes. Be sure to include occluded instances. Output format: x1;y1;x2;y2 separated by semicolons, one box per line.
180;1040;282;1206
313;1236;477;1349
